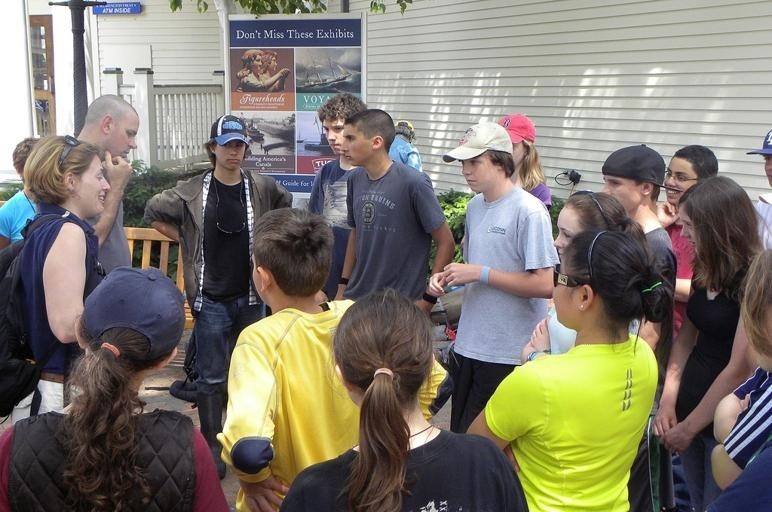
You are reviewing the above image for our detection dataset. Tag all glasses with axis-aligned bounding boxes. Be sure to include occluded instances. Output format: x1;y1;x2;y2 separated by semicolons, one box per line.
58;135;78;168
664;169;696;182
552;263;600;293
215;201;247;234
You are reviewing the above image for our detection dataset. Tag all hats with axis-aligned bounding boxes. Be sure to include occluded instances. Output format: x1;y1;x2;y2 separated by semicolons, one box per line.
395;121;418;141
442;122;514;162
602;143;679;192
499;115;536;147
82;267;186;361
209;115;248;147
747;130;772;154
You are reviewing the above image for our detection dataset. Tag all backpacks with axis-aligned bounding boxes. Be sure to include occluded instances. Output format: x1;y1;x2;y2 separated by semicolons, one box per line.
0;214;87;420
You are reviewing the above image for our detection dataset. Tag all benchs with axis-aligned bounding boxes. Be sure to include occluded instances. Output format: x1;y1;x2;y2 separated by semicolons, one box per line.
126;227;198;331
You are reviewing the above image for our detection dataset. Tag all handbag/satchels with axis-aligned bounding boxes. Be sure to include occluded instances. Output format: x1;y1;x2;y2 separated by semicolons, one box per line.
629;402;692;512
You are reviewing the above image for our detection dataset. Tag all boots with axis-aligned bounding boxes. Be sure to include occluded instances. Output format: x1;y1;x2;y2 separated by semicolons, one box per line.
197;393;225;479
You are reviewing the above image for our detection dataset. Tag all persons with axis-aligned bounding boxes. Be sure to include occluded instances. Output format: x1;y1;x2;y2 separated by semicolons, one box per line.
1;90;294;511
236;49;290;92
216;91;772;511
